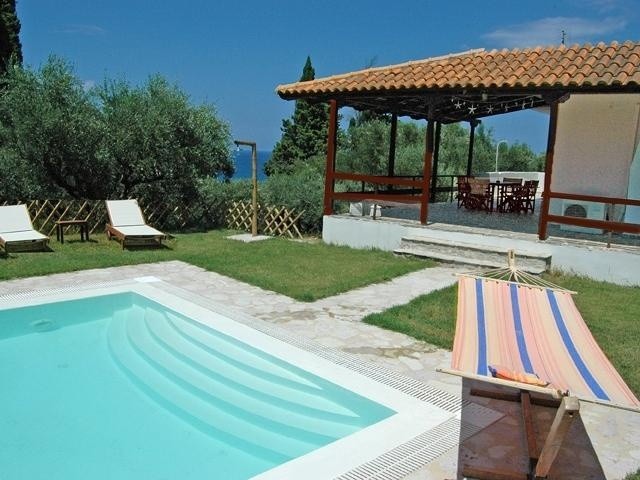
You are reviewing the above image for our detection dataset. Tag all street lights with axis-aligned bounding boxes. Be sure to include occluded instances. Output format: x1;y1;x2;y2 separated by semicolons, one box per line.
495;140;509;172
233;141;258;236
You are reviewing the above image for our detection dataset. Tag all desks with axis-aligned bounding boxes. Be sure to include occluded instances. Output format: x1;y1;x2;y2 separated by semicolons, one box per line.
55;220;88;244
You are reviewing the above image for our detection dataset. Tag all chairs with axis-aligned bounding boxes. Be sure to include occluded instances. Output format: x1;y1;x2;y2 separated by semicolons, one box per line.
455;173;540;215
104;197;174;251
0;203;50;258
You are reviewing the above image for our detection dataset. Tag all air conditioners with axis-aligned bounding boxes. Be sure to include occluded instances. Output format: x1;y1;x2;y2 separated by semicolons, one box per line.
558;198;608;236
